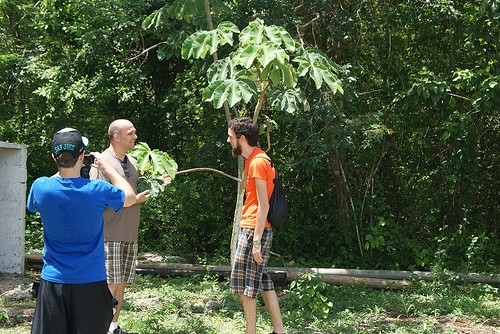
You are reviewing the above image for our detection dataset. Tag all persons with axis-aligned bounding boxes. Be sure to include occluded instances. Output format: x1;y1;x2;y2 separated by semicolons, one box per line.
27;127;137;333
227;117;284;334
89;119;151;334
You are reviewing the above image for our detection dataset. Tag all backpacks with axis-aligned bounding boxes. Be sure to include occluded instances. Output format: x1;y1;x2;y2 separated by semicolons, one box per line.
258;157;288;227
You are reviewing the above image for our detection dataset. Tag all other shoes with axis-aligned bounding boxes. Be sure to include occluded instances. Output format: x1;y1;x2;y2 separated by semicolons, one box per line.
107;326;137;334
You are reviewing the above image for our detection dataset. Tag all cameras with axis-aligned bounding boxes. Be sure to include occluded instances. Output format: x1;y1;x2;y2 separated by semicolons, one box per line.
80;155;94;179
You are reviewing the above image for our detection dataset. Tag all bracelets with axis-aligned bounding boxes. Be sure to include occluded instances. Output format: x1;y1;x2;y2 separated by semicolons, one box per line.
251;242;261;246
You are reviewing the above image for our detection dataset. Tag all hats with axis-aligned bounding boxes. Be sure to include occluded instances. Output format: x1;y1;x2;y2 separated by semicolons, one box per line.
52;128;89;158
